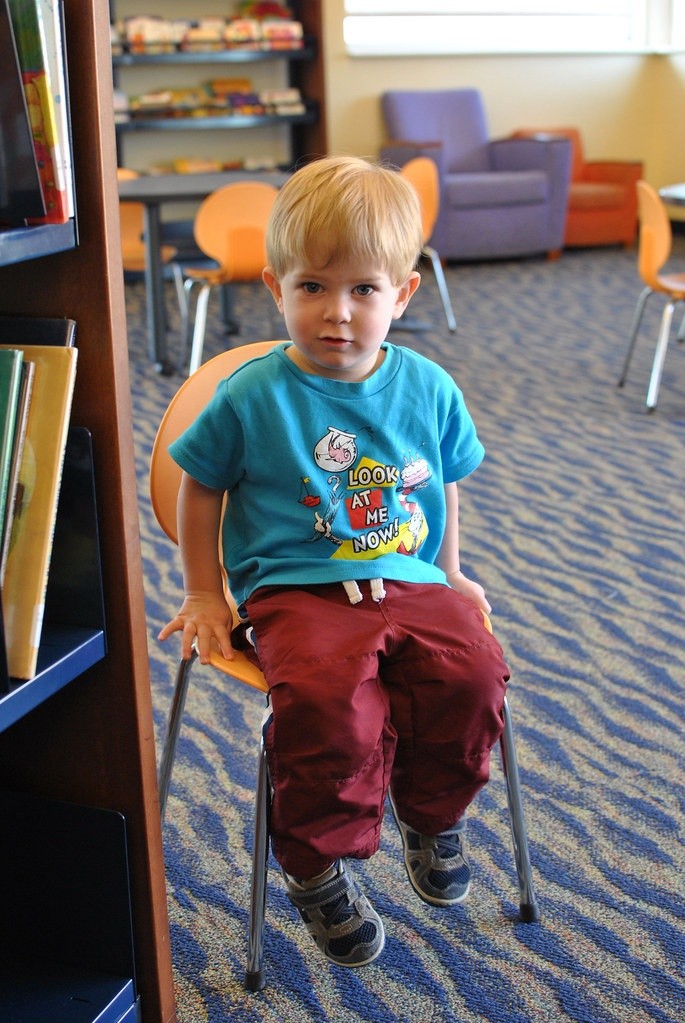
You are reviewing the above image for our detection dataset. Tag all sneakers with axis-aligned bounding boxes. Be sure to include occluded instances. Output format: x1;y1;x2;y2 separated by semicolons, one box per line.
280;855;385;968
387;785;471;906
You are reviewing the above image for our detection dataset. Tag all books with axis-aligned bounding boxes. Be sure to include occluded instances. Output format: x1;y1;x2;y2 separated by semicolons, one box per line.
0;0;75;226
0;317;79;690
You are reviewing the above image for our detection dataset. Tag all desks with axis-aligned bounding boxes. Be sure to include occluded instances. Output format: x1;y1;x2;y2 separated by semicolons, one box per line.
116;169;292;374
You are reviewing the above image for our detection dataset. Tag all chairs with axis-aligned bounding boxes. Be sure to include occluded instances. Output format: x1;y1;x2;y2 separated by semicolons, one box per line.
400;154;461;336
114;167;179;334
148;341;541;994
615;178;685;418
168;182;284;380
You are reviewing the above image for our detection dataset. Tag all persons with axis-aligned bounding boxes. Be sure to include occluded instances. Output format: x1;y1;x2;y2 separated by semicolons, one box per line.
157;159;510;966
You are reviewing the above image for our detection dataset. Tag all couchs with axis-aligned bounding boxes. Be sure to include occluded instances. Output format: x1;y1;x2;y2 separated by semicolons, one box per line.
508;128;645;264
379;86;571;270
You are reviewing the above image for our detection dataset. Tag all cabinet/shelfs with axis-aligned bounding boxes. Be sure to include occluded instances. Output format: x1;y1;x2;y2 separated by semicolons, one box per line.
109;0;346;286
0;0;179;1023
